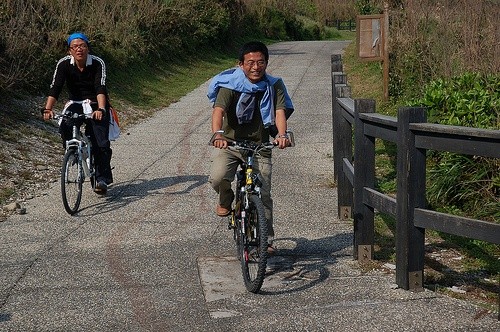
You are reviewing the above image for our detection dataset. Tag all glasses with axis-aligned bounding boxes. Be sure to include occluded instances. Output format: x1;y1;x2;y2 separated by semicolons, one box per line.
68;43;88;50
244;59;266;67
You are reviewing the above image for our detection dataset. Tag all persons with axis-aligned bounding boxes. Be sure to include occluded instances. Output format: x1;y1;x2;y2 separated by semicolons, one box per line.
44;33;111;192
209;42;291;257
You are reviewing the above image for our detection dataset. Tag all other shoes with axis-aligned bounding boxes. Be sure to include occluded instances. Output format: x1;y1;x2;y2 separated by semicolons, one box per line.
94;180;108;192
267;246;276;254
68;154;77;167
216;202;232;217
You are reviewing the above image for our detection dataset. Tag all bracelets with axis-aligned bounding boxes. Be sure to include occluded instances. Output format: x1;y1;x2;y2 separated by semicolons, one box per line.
276;133;289;139
97;108;105;117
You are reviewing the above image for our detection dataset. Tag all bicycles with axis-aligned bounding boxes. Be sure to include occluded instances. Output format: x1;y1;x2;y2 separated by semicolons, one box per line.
39;107;115;216
207;129;296;293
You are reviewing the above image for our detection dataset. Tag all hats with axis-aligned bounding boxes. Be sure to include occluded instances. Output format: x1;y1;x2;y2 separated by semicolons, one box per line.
67;33;88;47
